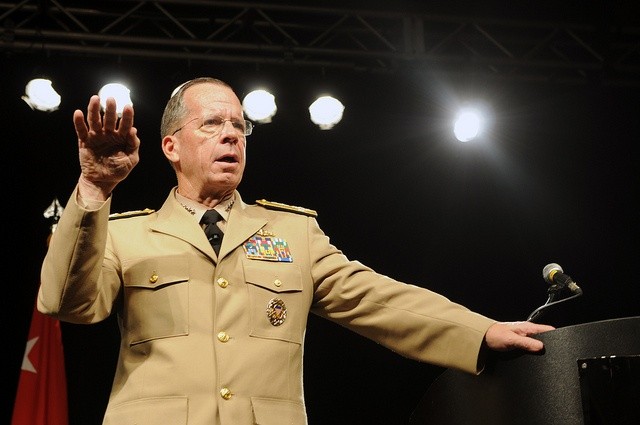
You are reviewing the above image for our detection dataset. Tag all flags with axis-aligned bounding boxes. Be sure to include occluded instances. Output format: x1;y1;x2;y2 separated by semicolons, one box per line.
10;282;70;425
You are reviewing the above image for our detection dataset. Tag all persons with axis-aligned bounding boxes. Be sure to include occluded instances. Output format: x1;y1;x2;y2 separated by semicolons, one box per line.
36;75;556;425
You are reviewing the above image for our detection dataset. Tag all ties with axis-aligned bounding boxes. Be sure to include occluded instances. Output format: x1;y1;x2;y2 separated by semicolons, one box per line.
200;209;224;257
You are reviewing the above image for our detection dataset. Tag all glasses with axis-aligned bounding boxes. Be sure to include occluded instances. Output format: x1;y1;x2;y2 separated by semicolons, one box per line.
172;114;255;136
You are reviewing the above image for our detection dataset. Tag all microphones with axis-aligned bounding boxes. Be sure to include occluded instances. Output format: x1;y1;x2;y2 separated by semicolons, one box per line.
543;264;582;299
